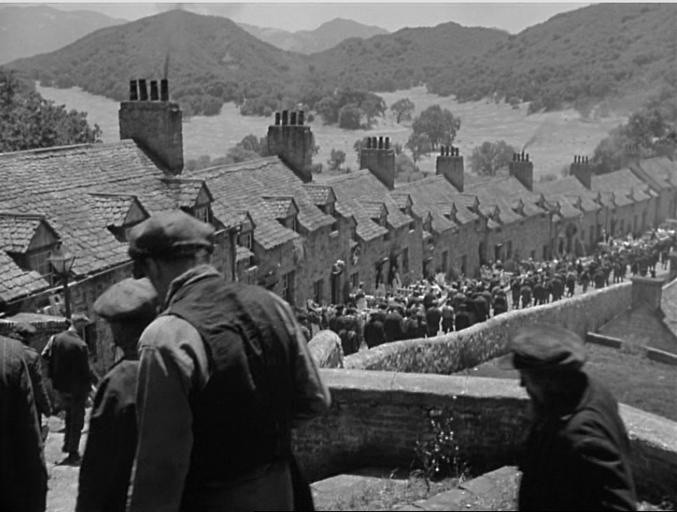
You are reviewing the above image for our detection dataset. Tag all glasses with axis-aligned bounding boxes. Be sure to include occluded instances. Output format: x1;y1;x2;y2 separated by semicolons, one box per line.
132;261;148;280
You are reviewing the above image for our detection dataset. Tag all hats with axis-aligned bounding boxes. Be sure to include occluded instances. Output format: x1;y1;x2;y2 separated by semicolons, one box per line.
129;209;215;255
71;313;90;323
514;322;587;375
13;321;37;336
93;277;159;320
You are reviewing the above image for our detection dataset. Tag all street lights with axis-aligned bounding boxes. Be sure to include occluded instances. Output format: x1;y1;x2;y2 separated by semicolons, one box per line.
47;239;75;319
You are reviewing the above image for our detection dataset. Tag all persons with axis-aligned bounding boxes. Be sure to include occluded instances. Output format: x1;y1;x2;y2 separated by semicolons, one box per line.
0;334;47;511
125;206;332;512
511;216;677;307
41;313;93;458
77;275;162;511
510;323;638;512
8;322;54;431
293;259;510;354
